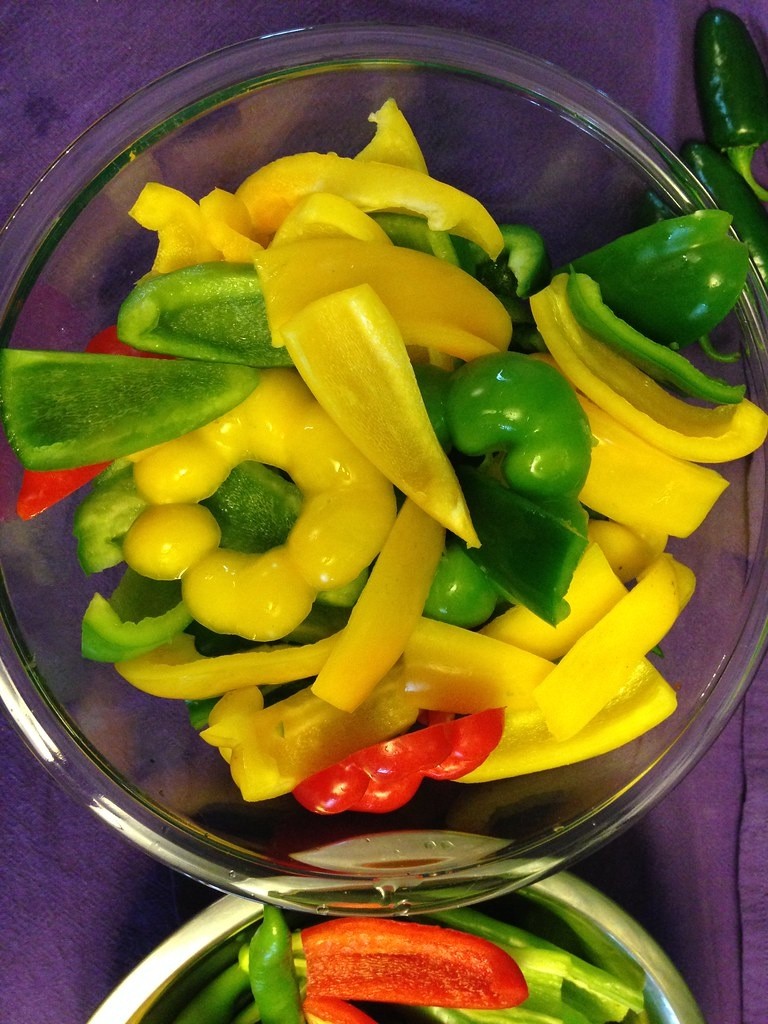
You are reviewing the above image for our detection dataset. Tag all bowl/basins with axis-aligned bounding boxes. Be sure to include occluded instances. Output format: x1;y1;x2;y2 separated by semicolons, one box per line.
1;21;768;919
81;830;703;1024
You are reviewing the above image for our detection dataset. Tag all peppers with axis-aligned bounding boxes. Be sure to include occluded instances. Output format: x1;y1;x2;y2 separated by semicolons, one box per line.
170;894;647;1024
0;8;768;818
